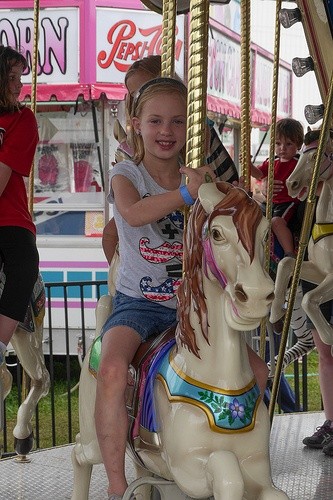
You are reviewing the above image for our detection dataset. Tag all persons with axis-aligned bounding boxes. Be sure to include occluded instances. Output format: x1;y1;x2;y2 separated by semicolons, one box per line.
0;45;40;366
102;56;239;266
251;118;303;259
300;124;332;454
94;77;269;500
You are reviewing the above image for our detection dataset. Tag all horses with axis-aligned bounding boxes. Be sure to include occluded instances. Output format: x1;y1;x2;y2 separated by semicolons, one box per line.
68;182;291;500
0;262;50;457
268;126;333;358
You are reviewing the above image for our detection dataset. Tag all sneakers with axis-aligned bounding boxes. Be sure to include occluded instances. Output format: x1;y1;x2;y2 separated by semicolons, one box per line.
322;438;333;457
302;420;333;449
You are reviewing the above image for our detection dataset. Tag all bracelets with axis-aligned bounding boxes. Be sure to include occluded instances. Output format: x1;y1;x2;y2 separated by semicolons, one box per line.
179;184;195;205
